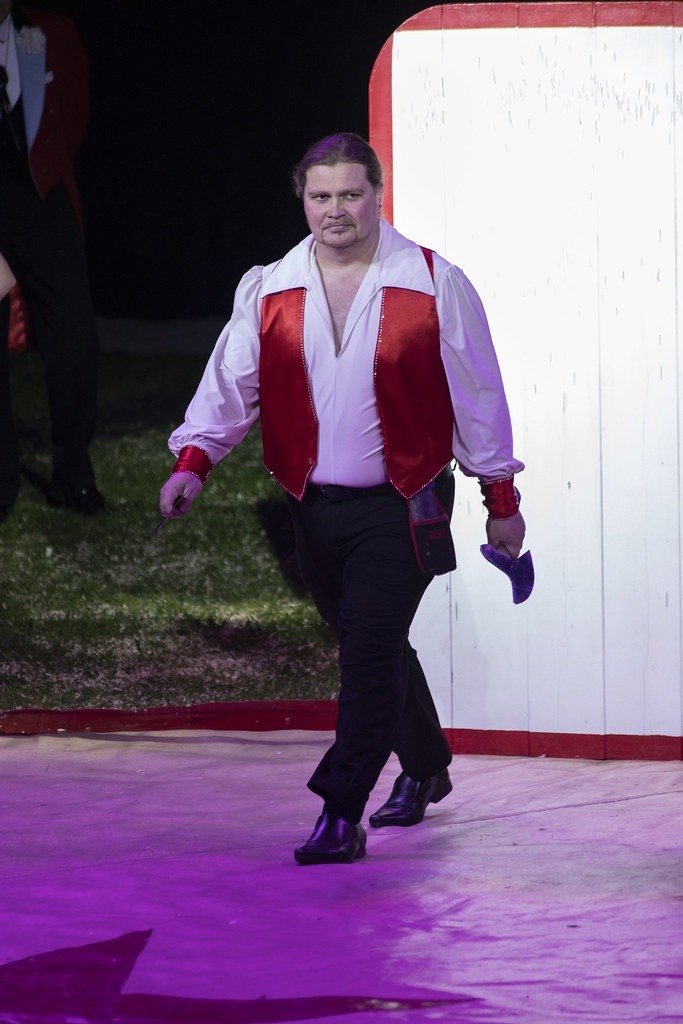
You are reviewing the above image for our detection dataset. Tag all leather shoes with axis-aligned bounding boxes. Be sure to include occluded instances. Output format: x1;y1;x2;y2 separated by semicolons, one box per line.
368;766;452;829
48;476;104;515
295;814;366;865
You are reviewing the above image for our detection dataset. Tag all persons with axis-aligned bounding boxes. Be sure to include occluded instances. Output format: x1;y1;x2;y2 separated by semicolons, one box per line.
159;134;527;866
0;0;105;522
